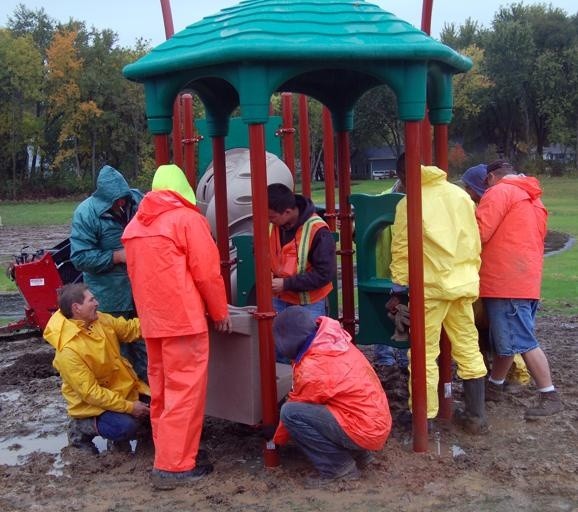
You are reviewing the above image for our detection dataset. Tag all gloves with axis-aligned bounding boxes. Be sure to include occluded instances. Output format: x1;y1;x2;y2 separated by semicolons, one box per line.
387;303;411;332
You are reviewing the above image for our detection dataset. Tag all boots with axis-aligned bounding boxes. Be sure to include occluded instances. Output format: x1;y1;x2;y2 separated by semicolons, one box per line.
67;417;99;445
455;378;487;433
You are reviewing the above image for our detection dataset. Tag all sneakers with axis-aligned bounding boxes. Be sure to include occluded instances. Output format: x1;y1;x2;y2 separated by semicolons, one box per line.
152;463;214;490
525;390;562;419
485;379;504;401
298;462;359;490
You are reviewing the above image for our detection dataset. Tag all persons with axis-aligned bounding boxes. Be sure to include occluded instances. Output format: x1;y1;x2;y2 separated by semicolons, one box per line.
374;153;565;434
121;164;233;489
44;283;151;448
70;165;151;405
267;183;337;364
273;304;392;488
